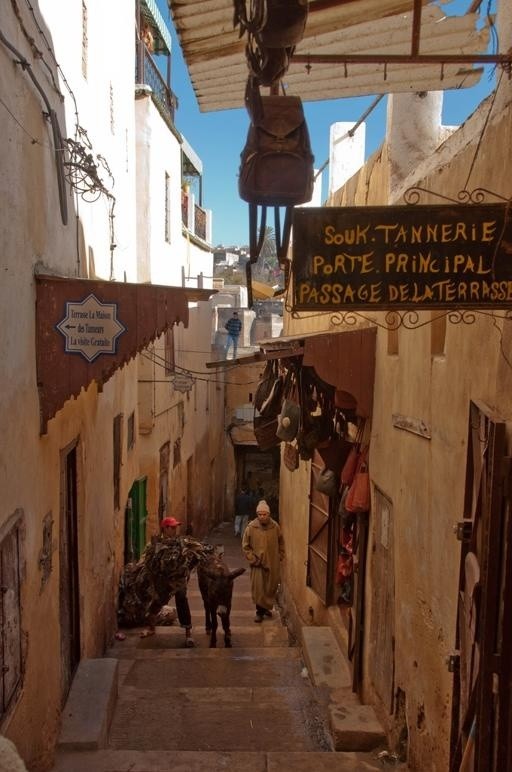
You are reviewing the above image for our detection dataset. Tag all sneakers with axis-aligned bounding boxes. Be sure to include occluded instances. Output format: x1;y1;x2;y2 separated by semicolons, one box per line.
139;629;156;637
185;637;194;647
254;615;264;623
263;609;272;616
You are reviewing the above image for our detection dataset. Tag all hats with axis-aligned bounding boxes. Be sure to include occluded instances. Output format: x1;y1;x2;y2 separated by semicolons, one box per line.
256;500;271;513
160;517;182;529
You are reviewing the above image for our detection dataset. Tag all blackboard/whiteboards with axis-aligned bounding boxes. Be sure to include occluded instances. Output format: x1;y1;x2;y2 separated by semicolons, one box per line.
293;202;511;311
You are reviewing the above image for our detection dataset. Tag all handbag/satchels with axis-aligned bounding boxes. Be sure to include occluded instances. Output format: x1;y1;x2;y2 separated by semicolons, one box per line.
318;449;369;517
341;523;360;553
252;366;333;470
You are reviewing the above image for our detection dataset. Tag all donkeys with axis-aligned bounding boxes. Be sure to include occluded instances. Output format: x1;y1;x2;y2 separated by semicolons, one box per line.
196;555;246;648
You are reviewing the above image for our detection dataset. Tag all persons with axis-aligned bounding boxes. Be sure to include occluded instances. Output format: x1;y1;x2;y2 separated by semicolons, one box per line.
138;517;194;648
242;500;287;623
224;312;242;358
234;489;251;538
